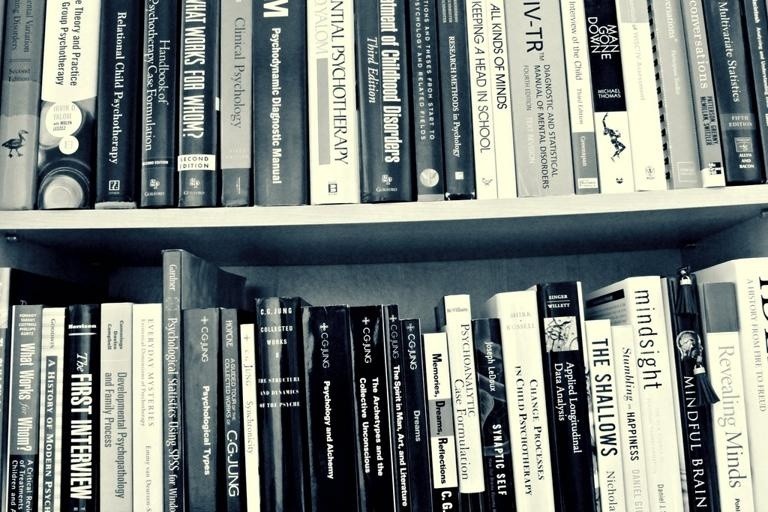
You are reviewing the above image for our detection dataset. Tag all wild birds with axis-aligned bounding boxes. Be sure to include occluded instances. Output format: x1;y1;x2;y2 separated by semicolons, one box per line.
1;130;29;158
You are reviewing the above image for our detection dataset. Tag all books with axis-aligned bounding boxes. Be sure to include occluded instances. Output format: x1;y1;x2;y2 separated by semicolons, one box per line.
1;247;767;512
0;0;768;212
